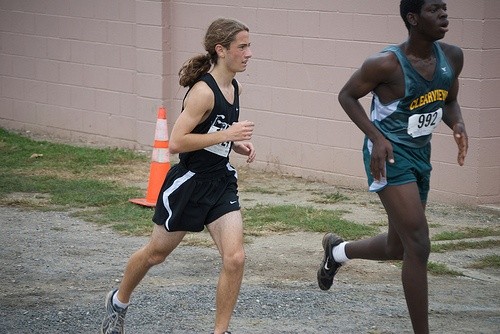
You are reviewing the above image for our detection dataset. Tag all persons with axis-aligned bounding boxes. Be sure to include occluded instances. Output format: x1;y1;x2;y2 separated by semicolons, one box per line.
316;0;469;334
99;18;254;334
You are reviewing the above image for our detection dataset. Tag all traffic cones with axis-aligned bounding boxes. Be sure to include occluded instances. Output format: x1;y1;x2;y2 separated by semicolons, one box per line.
129;105;168;207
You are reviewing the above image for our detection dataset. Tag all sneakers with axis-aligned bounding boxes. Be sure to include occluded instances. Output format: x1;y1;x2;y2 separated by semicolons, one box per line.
317;232;343;290
101;288;128;334
